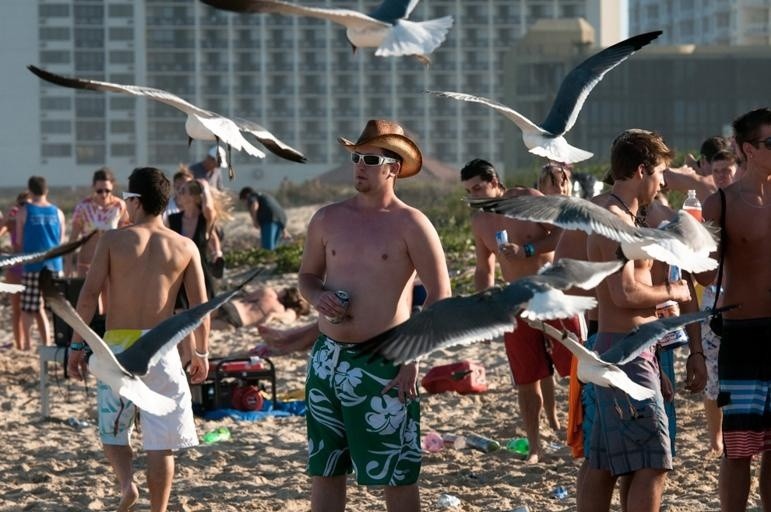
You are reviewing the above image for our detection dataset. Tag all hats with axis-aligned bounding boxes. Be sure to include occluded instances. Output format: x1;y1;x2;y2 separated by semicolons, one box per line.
336;115;428;180
208;145;230;169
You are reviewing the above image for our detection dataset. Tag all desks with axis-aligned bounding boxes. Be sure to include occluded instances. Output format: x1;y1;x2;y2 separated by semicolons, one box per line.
40;347;77;417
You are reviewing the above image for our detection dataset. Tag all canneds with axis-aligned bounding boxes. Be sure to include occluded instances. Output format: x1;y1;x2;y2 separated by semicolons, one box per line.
550;485;567;500
323;290;349;324
66;418;80;426
494;229;508;248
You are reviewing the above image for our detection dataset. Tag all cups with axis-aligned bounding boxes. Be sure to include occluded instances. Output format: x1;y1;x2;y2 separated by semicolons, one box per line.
441;432;468;450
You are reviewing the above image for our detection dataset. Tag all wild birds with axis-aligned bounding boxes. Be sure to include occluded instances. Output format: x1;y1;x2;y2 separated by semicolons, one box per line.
37;267;267;438
521;301;743;421
1;228;99;269
340;258;623;368
26;64;308;181
199;0;455;67
462;194;722;274
426;29;664;189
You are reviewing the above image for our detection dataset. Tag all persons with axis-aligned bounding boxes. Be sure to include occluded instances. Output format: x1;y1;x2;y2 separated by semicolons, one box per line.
240;188;292;251
297;119;453;512
66;167;211;512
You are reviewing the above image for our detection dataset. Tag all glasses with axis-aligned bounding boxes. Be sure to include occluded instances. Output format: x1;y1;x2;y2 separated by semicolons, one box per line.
96;188;114;195
122;190;142;202
347;149;401;171
744;136;771;150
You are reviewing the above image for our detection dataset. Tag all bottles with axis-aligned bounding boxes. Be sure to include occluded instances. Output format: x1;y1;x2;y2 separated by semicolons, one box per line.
684;188;702;225
507;438;530;456
202;427;230;443
460;430;500;454
